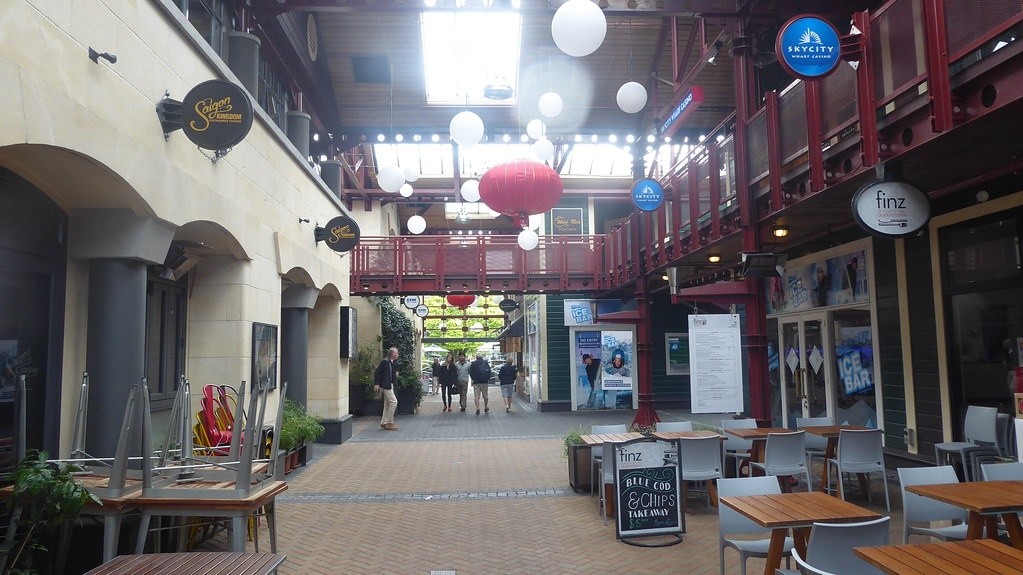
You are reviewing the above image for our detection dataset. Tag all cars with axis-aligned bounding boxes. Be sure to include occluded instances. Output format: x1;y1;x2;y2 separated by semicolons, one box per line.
486;359;507;382
419;364;434;386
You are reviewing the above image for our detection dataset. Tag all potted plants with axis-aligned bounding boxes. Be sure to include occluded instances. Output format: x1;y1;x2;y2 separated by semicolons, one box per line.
279;399;327;472
349;348;424;416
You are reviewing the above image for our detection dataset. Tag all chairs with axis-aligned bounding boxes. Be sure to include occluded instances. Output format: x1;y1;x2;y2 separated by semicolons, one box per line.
178;383;263;551
580;409;1023;575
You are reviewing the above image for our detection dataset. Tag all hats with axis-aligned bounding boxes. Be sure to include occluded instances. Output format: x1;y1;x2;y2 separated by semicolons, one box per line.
582;354;590;360
614;354;623;360
459;352;465;356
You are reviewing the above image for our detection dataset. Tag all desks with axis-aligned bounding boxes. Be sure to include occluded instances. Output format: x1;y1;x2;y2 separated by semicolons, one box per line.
581;432;645;526
0;503;137;575
724;427;798;481
1;373;183;502
651;430;728;515
905;480;1023;550
130;377;287;501
799;425;871;499
852;538;1023;575
718;492;881;575
85;552;287;575
134;485;293;575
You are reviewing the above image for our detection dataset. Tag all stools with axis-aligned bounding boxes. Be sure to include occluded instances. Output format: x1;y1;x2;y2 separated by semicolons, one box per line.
253;424;276;513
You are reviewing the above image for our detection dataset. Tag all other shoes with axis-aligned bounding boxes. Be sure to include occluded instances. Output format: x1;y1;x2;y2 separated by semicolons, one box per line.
476;409;480;414
460;405;467;412
485;407;489;412
381;424;390;430
387;423;398;429
505;404;512;412
448;408;452;412
443;406;447;412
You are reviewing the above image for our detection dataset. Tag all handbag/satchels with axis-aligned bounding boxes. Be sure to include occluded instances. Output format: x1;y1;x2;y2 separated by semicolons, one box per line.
451;385;459;395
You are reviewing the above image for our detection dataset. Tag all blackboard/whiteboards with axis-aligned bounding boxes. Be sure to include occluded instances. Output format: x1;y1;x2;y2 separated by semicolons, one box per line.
610;438;687;538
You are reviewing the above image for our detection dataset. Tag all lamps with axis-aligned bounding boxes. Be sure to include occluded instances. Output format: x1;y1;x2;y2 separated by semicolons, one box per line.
376;93;484;235
707;254;723;262
526;92;562;161
551;0;607;56
661;274;668;280
88;47;118;67
518;211;543;250
772;225;791;237
617;14;648;113
299;217;311;224
707;40;727;66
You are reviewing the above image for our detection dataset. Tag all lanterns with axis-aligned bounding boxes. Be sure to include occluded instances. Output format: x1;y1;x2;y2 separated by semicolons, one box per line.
478;159;563;227
446;295;475;309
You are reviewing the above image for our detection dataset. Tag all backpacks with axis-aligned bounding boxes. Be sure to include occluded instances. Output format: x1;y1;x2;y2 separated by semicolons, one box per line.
479;360;491;382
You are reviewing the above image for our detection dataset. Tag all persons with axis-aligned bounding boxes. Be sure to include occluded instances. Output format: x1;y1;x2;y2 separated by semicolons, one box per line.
431;358;440;395
468;352;491;414
769;257;858;310
605;348;632;377
373;347;399;431
498;357;516;413
455;352;471;412
582;353;601;390
437;354;458;412
